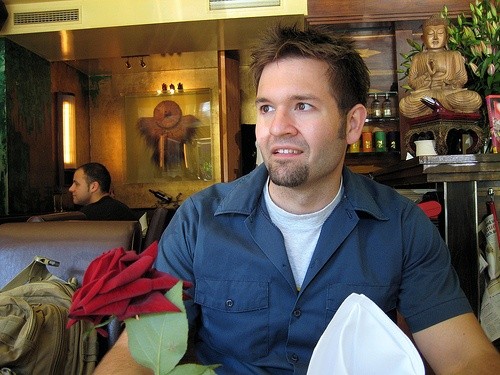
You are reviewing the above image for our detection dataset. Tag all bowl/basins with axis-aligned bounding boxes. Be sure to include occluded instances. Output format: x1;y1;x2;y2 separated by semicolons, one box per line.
414;140;438;157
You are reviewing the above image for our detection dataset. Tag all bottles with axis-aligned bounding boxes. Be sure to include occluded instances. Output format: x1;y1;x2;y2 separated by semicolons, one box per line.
482;141;489;154
382;93;392;117
148;189;172;203
162;83;167;91
177;83;183;92
170;84;174;90
371;94;382;118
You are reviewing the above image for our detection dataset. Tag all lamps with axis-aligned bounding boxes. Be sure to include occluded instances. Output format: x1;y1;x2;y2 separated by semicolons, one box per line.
125;57;132;69
56;91;76;170
139;56;146;68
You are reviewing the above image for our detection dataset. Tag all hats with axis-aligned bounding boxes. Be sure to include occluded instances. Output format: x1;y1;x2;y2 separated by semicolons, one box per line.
153;101;182;128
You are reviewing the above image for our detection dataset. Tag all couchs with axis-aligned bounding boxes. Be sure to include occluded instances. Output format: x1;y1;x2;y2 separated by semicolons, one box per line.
0;222;140;290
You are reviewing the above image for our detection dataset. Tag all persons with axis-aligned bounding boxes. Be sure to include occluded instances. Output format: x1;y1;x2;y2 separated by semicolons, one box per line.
69;162;141;221
91;21;500;375
399;14;482;116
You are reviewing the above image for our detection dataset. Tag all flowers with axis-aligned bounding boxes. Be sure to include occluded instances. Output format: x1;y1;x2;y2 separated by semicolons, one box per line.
66;240;222;375
396;0;500;100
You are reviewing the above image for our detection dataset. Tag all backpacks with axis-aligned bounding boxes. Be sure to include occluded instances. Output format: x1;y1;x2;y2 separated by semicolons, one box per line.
0;255;96;375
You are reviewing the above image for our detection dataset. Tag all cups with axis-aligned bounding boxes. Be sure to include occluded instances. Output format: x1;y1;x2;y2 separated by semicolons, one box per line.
349;131;399;152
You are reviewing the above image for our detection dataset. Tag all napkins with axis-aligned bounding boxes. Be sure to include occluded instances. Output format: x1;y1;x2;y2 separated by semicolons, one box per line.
305;292;424;375
